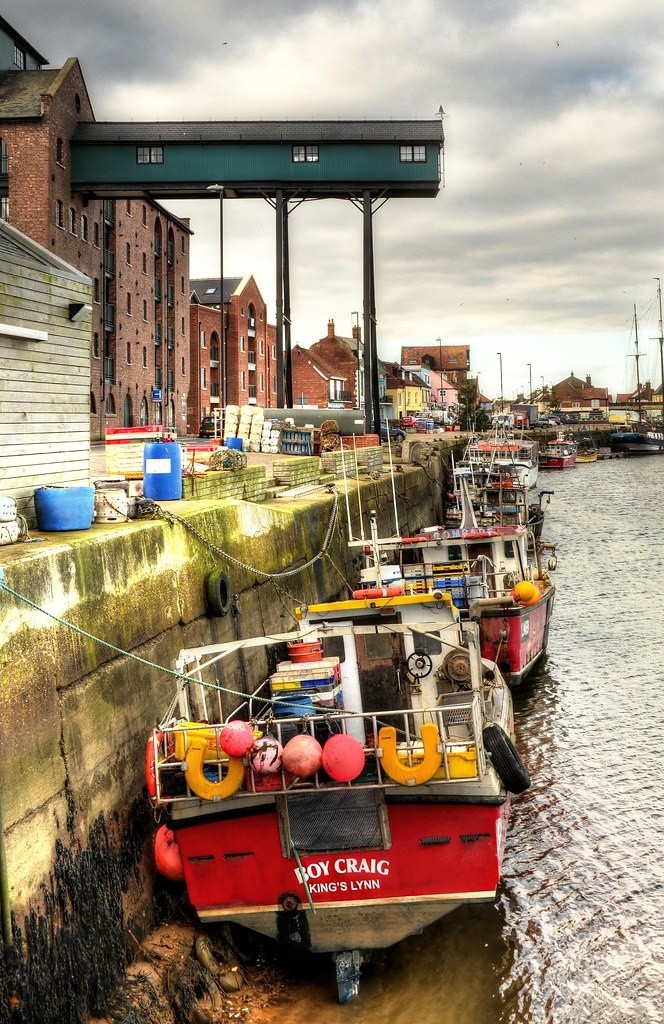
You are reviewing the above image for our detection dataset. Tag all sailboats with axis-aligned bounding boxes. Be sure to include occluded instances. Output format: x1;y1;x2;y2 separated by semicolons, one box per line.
608;277;664;456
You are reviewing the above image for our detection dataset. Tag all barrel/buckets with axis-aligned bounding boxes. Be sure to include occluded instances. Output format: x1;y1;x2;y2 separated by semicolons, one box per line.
258;695;317;750
287;641;324;663
416;418;452;434
242;440;250;451
34;486;95;531
143;442;183;501
225;437;242;453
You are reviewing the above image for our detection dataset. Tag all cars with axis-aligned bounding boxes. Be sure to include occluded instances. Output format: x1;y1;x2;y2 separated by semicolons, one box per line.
198;416;222;438
400;416;435;427
492;405;571;430
370;420;407;443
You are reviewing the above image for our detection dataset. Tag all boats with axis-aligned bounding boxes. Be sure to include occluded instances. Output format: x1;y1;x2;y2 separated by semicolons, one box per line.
147;588;517;1007
443;404;601;554
337;416;556;689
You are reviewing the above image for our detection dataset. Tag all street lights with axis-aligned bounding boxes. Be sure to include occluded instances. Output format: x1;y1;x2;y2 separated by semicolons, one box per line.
206;183;226;436
435;337;445;427
351;309;362;408
461;395;468;438
496;353;504;413
540;376;545;414
526;363;533;404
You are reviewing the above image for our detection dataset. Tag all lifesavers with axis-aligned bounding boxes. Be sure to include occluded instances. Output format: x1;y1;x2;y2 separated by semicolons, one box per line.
207;570;231;617
402;537;426;544
353;587;404;599
378;724;443;787
145;732;175;809
492;482;512;488
183;739;245;803
464;532;498;539
483;722;531;795
